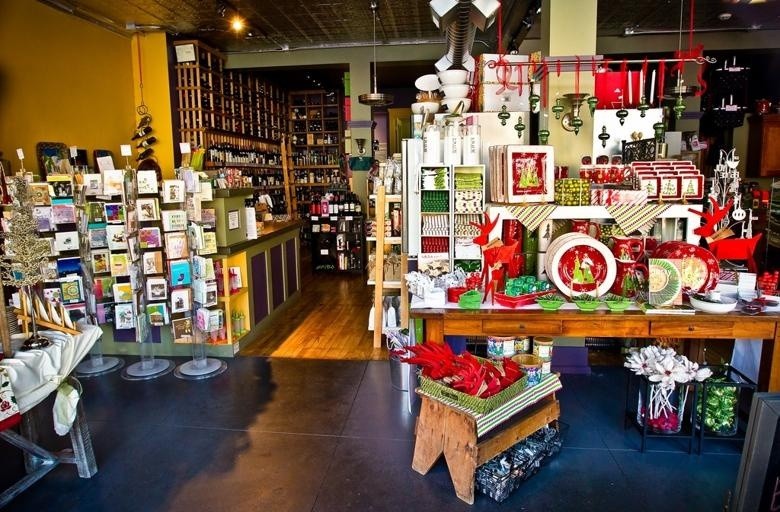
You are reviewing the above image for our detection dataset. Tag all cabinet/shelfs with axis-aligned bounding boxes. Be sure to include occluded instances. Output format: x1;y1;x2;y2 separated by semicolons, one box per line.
363;55;530;347
703;177;780;256
481;203;703;282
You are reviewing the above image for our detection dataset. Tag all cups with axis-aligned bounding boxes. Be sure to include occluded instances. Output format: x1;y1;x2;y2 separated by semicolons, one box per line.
579;164;625;205
487;334;554;387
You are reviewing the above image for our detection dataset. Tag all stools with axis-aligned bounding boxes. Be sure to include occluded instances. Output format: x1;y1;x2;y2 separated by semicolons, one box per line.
411;369;563;505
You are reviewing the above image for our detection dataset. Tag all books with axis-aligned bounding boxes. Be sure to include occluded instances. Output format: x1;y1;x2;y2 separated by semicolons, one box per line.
2;167;223;341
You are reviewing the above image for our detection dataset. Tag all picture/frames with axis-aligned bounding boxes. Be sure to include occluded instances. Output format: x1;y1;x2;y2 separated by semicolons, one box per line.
36;142;70;183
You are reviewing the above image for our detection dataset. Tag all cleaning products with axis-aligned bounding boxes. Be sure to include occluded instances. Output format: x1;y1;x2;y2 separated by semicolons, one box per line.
423;121;479;164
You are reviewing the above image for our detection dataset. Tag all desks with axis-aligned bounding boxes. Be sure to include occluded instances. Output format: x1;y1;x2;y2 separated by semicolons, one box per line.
0;323;103;507
408;308;780;445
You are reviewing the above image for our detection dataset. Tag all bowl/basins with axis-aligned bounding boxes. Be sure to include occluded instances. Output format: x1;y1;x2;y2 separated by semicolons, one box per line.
411;69;471;114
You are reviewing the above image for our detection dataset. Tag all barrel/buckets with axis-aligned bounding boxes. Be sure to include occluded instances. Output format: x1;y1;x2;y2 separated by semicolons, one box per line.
390;356;409;391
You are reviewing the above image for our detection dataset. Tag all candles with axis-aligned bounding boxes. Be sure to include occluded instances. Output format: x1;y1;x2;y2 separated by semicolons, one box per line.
16;148;24;160
70;145;78;157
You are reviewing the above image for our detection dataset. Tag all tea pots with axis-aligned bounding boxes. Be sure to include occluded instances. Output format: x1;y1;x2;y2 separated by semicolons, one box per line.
566;219;602;240
607;236;648;299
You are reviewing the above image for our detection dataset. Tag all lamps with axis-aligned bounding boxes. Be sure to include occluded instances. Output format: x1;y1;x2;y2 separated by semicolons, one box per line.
358;0;394;108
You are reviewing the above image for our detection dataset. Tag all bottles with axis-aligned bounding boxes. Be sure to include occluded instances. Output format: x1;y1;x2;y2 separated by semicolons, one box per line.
425;124;480;165
208;139;362;218
130;114;157;162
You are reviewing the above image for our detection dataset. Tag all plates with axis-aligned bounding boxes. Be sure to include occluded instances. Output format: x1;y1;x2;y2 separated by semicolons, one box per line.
493;289;558;308
652;240;739;315
544;232;617;299
